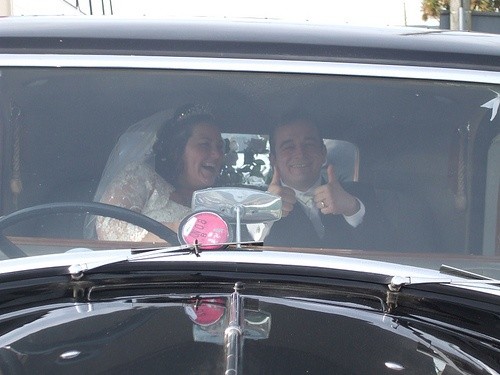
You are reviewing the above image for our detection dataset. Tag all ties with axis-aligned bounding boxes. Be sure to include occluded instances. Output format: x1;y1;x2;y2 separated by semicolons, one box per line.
298;194;325;240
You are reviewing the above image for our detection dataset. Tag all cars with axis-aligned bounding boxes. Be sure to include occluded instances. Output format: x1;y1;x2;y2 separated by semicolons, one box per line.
1;14;500;375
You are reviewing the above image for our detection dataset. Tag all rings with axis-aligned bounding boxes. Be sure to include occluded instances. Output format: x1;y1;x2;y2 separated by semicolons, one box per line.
321;201;326;209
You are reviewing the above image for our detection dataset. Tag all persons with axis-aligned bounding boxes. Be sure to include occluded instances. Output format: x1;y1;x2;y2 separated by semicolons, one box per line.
84;103;225;243
231;114;402;249
